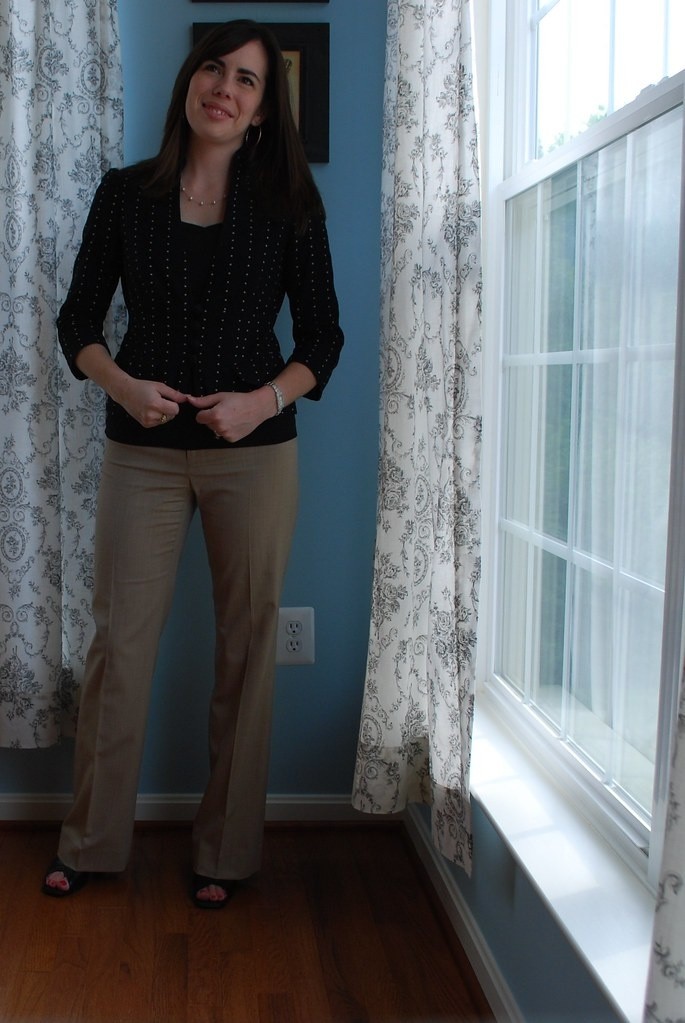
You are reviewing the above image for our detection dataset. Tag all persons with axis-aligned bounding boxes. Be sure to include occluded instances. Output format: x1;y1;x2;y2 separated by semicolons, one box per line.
40;19;344;910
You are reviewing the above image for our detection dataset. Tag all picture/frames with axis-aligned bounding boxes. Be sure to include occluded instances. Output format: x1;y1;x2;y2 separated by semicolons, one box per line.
193;20;334;165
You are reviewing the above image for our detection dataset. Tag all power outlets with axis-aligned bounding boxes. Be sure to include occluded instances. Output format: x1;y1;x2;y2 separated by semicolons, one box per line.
275;607;315;665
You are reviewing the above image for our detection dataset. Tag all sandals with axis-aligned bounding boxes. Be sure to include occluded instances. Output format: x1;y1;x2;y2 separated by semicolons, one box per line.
41;854;89;897
191;876;242;910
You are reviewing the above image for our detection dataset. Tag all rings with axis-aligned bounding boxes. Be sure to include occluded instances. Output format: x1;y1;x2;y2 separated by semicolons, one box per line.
214;431;222;437
160;413;168;423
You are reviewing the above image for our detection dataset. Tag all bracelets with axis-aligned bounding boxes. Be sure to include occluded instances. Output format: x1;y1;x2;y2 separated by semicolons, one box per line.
264;381;284;416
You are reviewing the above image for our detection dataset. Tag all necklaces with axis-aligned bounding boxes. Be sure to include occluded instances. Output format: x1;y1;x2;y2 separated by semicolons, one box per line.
180;176;228;206
